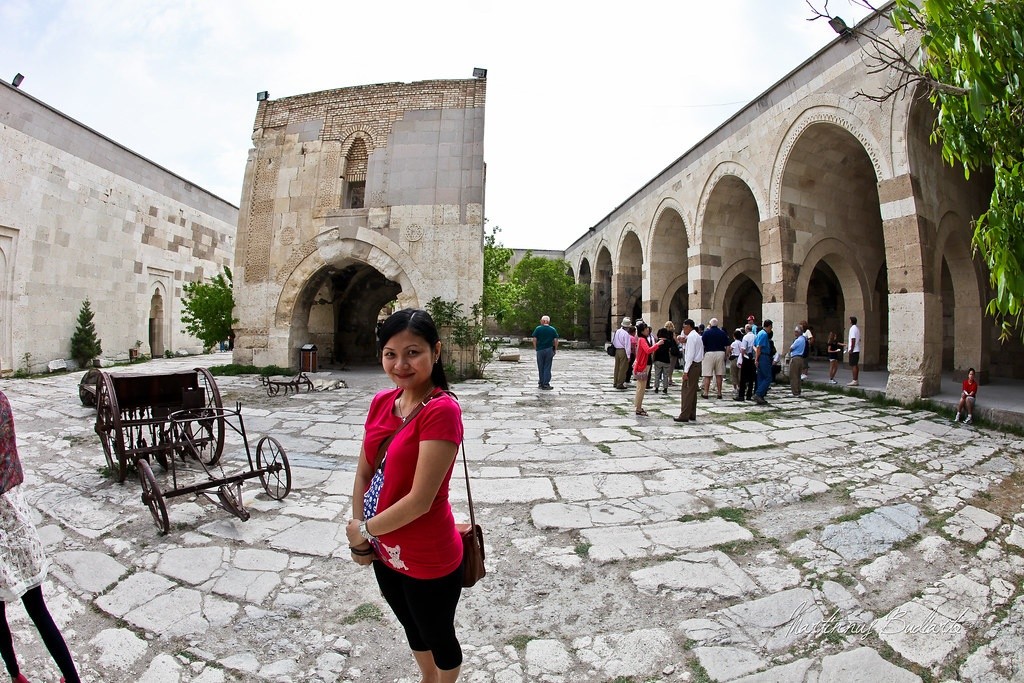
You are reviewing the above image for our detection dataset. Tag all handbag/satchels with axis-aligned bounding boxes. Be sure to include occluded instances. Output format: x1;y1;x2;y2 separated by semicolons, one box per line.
455;524;485;588
607;344;616;357
633;371;648;380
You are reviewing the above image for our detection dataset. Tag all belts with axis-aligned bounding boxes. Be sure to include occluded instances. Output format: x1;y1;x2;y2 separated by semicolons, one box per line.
790;355;803;358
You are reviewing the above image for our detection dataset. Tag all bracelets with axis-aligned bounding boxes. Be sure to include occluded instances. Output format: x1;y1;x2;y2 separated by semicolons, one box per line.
351;547;373;556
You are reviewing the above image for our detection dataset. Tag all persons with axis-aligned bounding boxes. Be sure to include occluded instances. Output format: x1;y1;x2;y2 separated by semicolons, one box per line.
677;335;688;344
847;316;861;386
788;325;805;398
753;319;774;404
828;331;847;383
702;318;730;399
694;324;705;335
0;391;82;683
612;318;631;389
346;305;463;683
674;319;704;422
654;320;679;394
746;315;758;334
634;323;664;416
772;346;781;382
626;326;637;382
800;321;814;374
730;324;757;402
532;316;559;390
954;368;977;424
646;326;657;388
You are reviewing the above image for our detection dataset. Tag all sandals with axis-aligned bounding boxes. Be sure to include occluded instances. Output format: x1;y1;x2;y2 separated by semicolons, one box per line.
636;409;648;416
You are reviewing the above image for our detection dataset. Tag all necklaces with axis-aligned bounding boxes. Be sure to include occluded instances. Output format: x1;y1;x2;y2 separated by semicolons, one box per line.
399;391;431;423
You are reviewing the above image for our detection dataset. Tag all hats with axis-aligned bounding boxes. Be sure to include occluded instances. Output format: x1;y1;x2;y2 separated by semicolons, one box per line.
747;315;755;320
621;318;631;327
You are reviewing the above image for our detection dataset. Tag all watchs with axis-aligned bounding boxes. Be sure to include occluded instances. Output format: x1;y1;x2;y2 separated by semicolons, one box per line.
360;521;376;540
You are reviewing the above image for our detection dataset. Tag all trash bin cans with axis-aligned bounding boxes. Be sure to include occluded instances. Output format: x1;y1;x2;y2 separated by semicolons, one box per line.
300;344;318;373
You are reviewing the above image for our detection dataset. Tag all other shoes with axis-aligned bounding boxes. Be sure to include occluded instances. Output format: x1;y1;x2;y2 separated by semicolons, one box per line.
964;418;971;424
614;378;716;394
538;384;553;390
11;674;30;683
753;394;764;402
60;676;66;683
848;380;859;386
732;396;744;401
829;379;837;384
788;393;799;398
674;417;688;422
717;395;722;399
701;393;708;399
955;417;960;421
757;401;768;405
800;374;807;379
746;398;753;400
689;417;696;421
